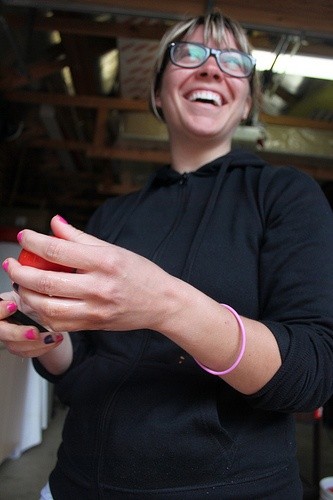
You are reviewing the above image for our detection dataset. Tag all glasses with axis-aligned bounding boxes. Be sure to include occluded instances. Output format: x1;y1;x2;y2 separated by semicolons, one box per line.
167;41;254;79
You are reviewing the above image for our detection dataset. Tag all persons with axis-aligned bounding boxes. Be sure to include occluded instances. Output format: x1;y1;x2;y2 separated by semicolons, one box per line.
0;10;333;500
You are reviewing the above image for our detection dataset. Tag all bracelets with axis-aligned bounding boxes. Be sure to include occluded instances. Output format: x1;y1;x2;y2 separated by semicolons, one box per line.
195;304;246;375
